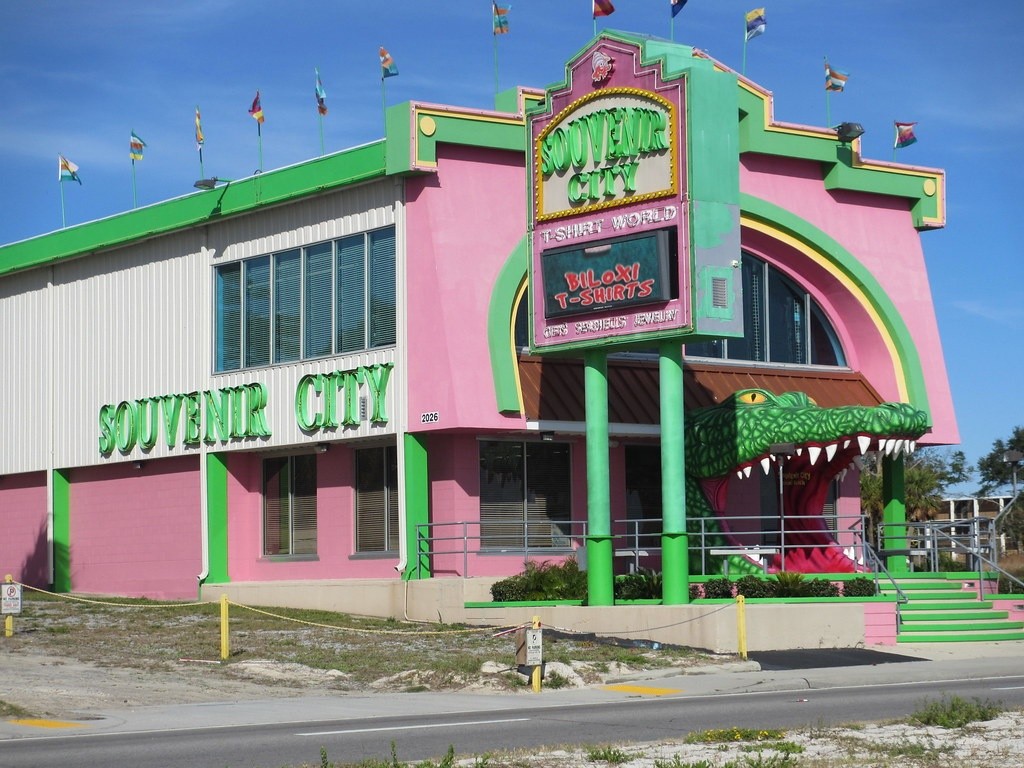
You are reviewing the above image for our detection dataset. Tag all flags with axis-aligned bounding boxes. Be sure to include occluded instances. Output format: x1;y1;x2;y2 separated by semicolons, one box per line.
825;62;850;93
493;3;509;35
248;91;264;124
59;155;82;186
671;0;687;18
196;106;205;151
893;122;918;149
744;8;766;43
593;0;615;17
315;67;329;116
130;132;147;160
380;46;399;78
